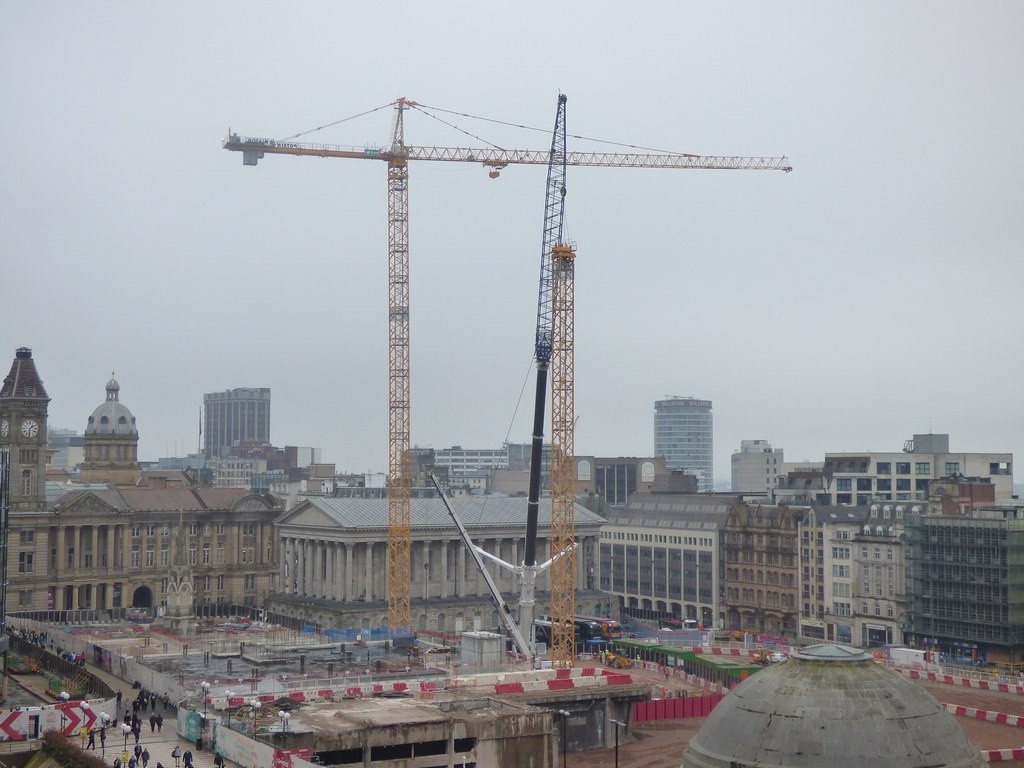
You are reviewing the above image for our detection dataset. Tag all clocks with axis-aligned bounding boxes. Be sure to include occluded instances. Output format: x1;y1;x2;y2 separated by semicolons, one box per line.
21;419;39;439
1;420;9;439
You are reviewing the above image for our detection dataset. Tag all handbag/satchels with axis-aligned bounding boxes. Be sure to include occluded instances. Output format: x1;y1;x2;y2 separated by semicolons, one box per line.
171;751;175;757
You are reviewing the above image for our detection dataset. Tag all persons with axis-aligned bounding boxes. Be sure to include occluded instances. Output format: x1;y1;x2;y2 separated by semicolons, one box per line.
86;687;224;768
874;649;887;664
3;623;87;666
594;650;669;679
